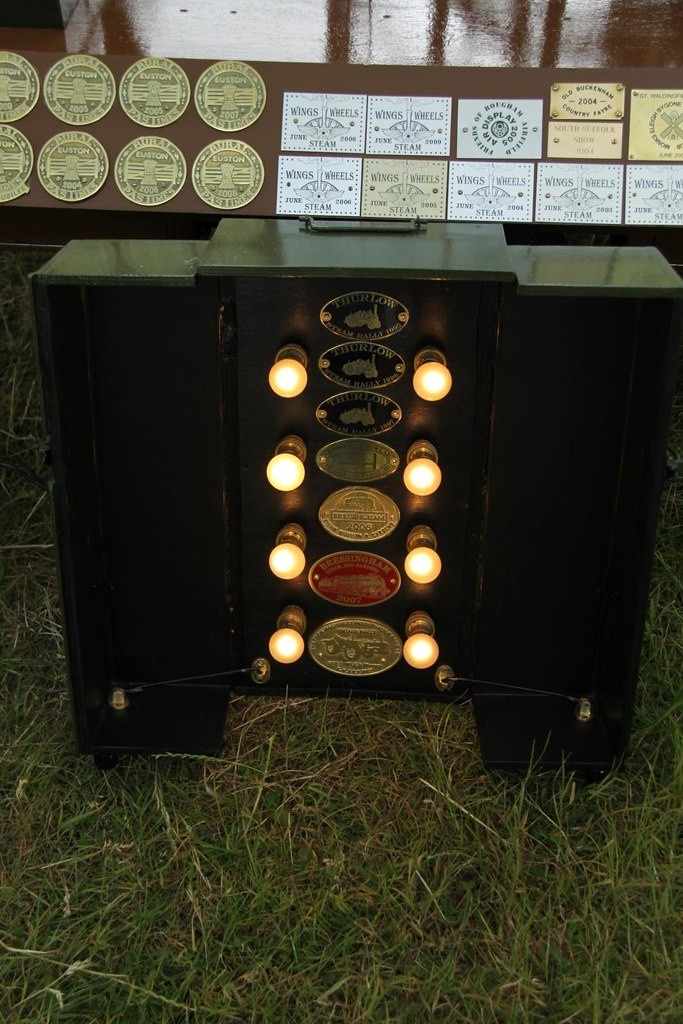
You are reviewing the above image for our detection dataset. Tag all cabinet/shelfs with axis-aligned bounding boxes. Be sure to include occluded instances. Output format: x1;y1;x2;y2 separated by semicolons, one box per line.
27;216;683;774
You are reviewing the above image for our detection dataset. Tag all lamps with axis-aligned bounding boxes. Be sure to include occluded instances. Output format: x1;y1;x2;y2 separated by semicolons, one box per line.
268;605;307;665
403;439;442;497
267;523;308;580
413;348;452;402
267;342;309;398
266;435;307;494
404;524;441;585
403;610;439;670
